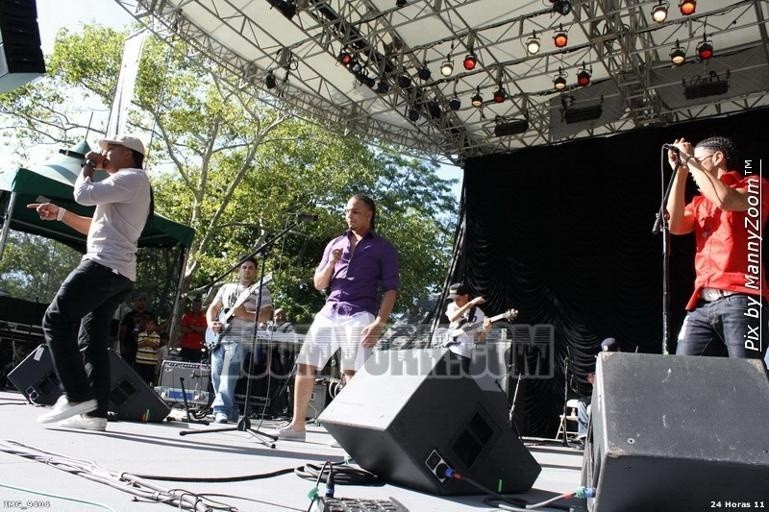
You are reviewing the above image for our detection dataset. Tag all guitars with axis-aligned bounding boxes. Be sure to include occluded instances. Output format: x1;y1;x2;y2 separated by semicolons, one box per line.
205;273;272;351
430;309;518;349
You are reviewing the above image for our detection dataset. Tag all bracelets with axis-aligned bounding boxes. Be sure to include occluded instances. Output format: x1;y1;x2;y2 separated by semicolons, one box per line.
685;155;695;164
377;308;388;320
55;206;66;222
80;158;96;171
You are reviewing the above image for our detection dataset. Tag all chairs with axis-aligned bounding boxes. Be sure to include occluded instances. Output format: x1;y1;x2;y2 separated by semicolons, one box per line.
555;399;587;439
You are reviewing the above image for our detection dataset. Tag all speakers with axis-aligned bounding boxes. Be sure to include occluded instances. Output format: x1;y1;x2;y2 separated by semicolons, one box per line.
316;348;542;495
6;343;64;406
0;0;46;92
232;349;282;416
84;346;171;423
158;359;213;406
580;352;769;512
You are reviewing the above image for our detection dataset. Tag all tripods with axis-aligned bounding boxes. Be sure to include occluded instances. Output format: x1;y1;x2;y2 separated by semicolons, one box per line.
179;245;277;448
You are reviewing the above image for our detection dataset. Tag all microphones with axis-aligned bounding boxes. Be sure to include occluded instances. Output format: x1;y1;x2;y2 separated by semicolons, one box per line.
668;144;680;156
59;148;86;159
289;213;318;222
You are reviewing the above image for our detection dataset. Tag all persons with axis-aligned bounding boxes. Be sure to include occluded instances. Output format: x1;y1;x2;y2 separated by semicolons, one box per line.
24;131;153;433
204;254;275;425
248;307;295;422
107;291;208;389
272;194;402;442
661;134;769;359
441;282;495;378
569;336;622;453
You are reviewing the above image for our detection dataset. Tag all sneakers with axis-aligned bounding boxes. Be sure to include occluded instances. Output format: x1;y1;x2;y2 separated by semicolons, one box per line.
57;414;108;431
39;395;97;423
216;413;228;423
270;426;307;442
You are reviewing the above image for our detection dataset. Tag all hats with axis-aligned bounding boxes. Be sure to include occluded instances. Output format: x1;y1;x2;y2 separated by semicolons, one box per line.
446;283;467;299
600;337;620;356
99;135;145;155
